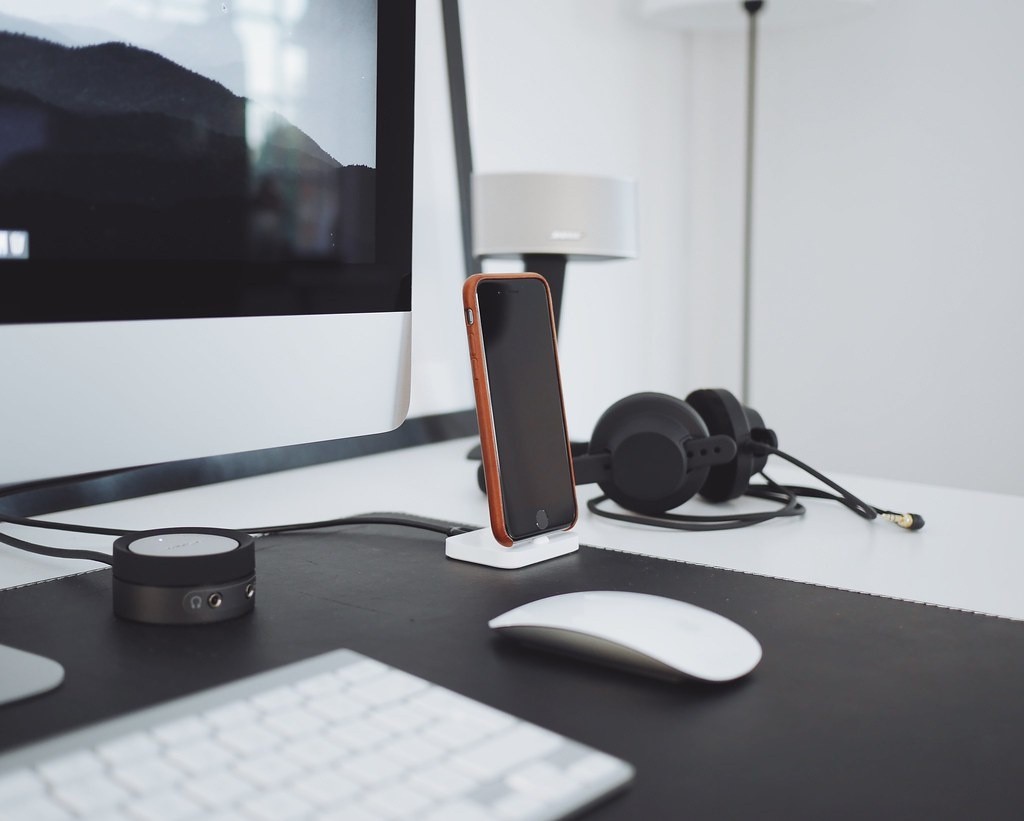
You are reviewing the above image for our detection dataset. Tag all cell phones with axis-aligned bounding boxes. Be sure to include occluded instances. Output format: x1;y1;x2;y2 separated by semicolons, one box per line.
462;272;578;547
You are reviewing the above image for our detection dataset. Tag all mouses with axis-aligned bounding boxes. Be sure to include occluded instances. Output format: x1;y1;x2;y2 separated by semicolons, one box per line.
489;591;764;685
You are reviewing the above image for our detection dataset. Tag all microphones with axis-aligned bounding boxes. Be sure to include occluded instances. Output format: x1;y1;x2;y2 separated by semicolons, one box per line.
747;441;878;520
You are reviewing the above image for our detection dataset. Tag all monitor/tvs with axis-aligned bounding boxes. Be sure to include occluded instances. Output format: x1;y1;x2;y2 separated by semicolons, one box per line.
0;0;417;706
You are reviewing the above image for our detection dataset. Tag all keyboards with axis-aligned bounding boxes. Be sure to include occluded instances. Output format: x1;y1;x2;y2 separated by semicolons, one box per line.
0;644;636;821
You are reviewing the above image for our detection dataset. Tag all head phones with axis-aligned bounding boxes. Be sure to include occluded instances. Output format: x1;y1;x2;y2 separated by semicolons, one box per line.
478;388;780;515
